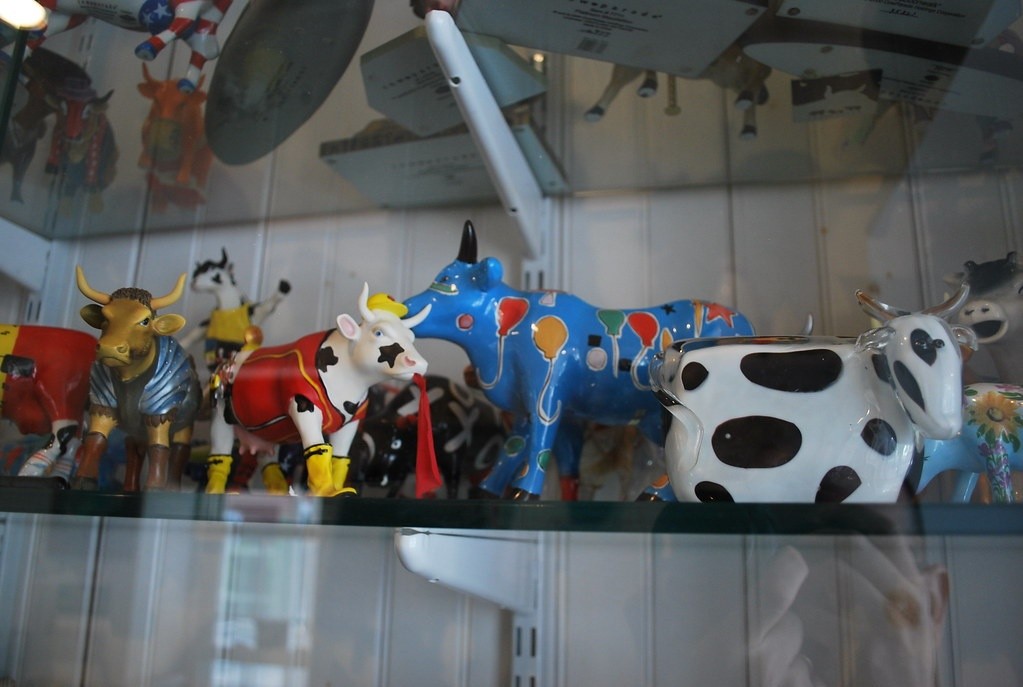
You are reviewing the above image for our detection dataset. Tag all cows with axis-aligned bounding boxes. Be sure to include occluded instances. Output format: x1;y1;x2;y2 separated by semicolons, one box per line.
398;220;816;507
1;324;101;489
582;43;772;143
201;279;435;499
943;251;1023;385
136;61;215;215
644;280;980;505
69;264;203;495
278;372;505;500
43;87;121;214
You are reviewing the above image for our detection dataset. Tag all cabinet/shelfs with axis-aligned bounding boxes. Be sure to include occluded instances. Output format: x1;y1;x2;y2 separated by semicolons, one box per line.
0;0;1023;687
